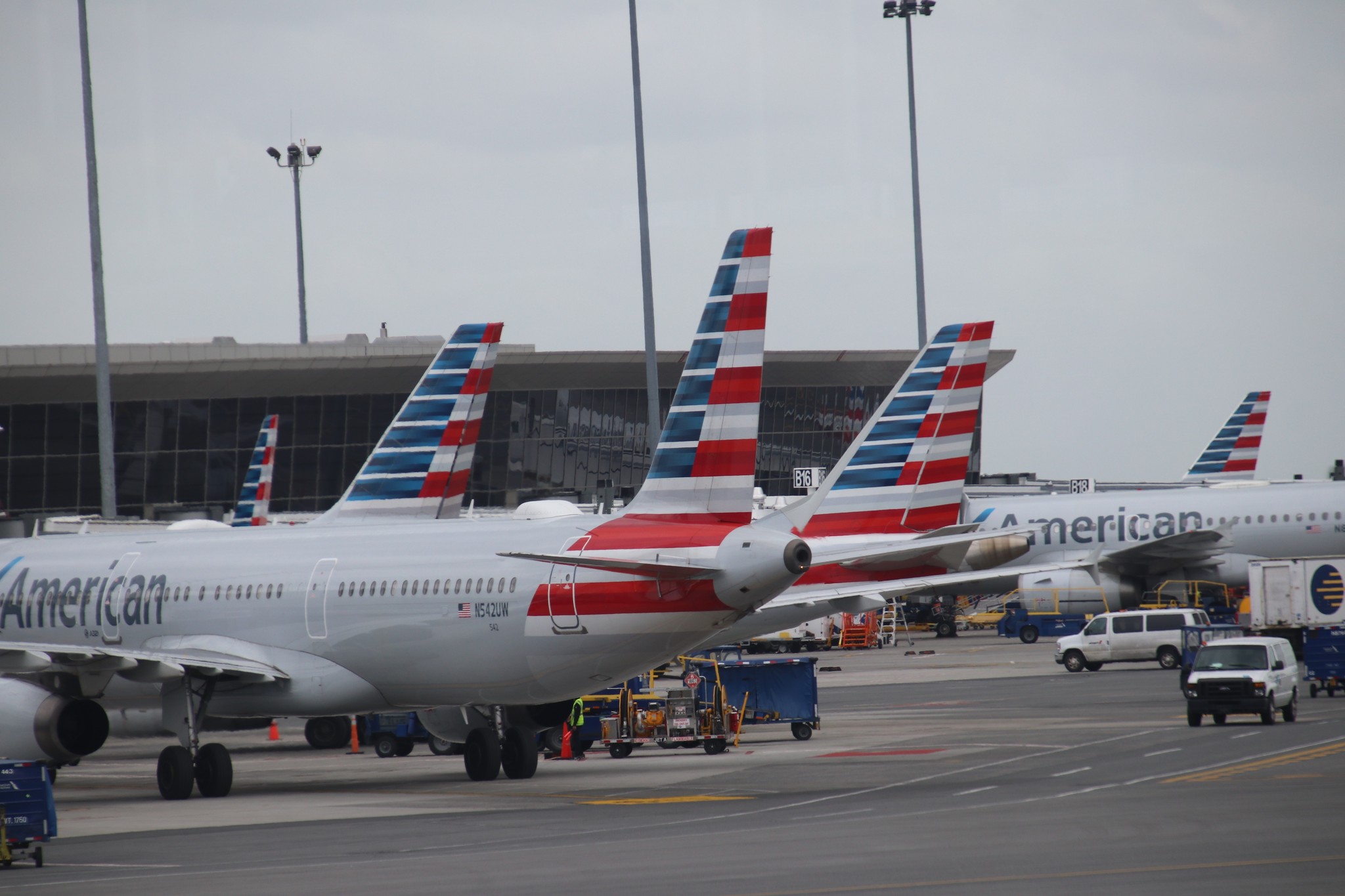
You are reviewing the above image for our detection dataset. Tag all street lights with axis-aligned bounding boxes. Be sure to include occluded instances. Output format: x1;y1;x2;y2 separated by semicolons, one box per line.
882;1;936;356
266;144;322;345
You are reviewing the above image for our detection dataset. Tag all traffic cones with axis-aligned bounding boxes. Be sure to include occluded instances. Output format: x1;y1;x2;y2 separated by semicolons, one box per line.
266;719;283;741
556;722;576;760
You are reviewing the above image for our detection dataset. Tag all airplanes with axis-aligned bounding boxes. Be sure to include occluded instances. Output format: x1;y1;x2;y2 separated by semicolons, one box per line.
1;227;1345;799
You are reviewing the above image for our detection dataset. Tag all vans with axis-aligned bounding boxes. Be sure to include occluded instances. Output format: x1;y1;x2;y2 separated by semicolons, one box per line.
1183;636;1299;727
1054;607;1213;673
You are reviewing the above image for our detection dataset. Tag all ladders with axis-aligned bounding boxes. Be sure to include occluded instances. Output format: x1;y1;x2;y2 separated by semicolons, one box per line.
879;603;913;647
